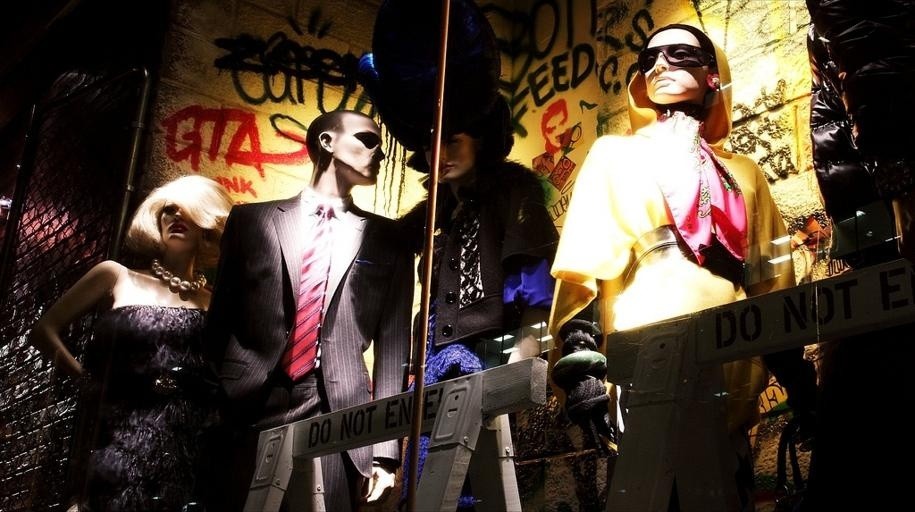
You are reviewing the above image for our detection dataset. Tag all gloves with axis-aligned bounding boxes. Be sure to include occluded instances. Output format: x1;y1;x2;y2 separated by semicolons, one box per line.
564;374;612;427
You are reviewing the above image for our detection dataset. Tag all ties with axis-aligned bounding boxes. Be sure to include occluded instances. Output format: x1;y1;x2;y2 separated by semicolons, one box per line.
280;203;333;380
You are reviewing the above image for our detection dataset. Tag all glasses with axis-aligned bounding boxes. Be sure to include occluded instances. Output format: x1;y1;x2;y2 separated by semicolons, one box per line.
638;45;712;74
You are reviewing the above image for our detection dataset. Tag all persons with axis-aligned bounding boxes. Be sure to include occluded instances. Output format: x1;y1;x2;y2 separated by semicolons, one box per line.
29;172;236;512
545;22;820;512
802;0;915;512
395;99;611;512
199;109;412;512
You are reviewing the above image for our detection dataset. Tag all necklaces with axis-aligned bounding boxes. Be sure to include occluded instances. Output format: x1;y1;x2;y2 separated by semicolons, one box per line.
148;258;207;292
